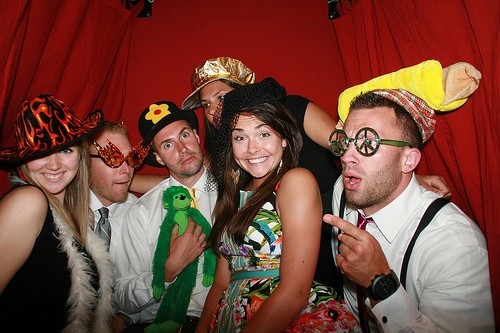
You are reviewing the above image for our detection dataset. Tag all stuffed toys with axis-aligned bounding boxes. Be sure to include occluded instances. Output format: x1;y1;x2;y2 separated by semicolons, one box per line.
145;185;216;333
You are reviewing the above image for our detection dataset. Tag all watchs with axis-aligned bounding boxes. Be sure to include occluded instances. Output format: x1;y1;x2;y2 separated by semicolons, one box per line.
366;270;400;300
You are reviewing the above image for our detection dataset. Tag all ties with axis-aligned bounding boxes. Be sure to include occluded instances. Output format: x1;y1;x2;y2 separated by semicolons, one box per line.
94;207;111;253
357;212;374;230
187;188;196;208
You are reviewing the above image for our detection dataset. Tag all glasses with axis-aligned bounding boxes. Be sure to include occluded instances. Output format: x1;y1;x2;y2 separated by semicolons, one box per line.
87;138;154;169
327;129;413;157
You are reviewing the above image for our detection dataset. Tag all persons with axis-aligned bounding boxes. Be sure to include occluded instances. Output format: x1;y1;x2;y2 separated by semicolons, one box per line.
0;94;167;333
113;101;217;333
323;90;495;333
181;56;451;297
86;121;140;259
195;77;363;333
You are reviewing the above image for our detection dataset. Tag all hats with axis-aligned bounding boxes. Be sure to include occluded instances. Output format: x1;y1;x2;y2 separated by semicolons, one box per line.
369;86;436;143
135;101;199;167
180;57;256;110
0;93;104;170
